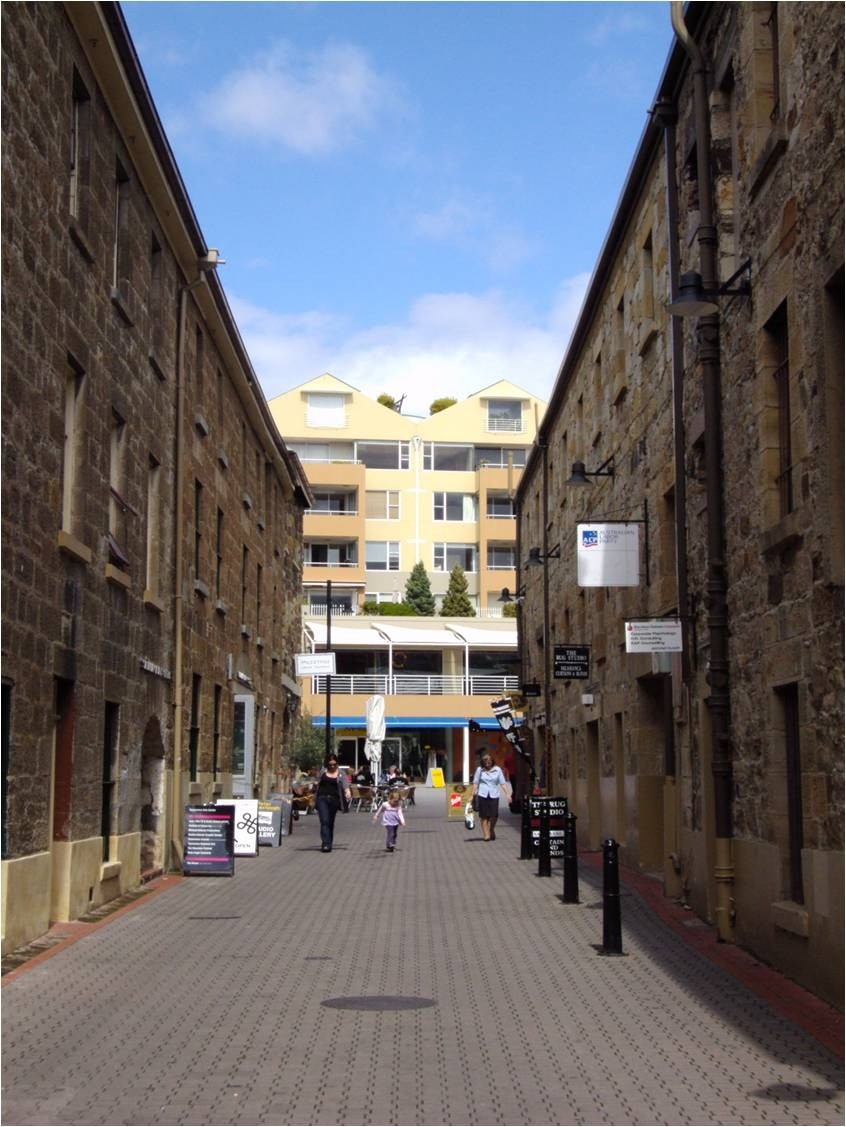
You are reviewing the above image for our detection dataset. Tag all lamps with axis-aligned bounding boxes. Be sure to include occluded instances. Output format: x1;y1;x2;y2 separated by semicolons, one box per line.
522;677;544;697
522;543;565;565
562;454;616;487
497;588;526;603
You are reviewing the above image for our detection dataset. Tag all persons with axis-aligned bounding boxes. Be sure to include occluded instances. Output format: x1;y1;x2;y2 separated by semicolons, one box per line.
504;748;515;807
471;753;513;841
373;792;406;852
309;754;351;852
356;765;410;811
407;738;424;779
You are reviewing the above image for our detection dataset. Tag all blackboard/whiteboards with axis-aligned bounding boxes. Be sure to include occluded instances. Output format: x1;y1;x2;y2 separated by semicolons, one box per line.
183;805;234;874
256;800;283;845
266;792;293;836
527;797;568;859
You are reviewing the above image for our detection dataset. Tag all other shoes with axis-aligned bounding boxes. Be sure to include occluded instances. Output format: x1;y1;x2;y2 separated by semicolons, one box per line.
490;830;495;840
391;846;395;851
321;842;331;853
387;844;396;849
484;835;490;841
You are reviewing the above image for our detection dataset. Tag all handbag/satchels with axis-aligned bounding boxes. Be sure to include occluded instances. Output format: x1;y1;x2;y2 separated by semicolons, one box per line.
473;794;480;812
465;801;475;829
339;774;349;813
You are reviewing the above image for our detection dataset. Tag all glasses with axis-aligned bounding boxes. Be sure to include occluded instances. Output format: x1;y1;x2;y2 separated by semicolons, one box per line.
329;764;337;767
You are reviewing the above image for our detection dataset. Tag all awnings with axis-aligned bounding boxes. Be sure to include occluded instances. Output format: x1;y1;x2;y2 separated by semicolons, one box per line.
311;715;469;729
470;717;525;733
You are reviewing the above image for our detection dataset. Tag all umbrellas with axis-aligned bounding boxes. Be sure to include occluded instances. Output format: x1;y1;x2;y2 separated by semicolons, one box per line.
363;695;387;811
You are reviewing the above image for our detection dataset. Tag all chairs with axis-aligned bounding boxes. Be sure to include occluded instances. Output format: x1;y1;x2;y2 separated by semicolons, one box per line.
348;785;415;814
